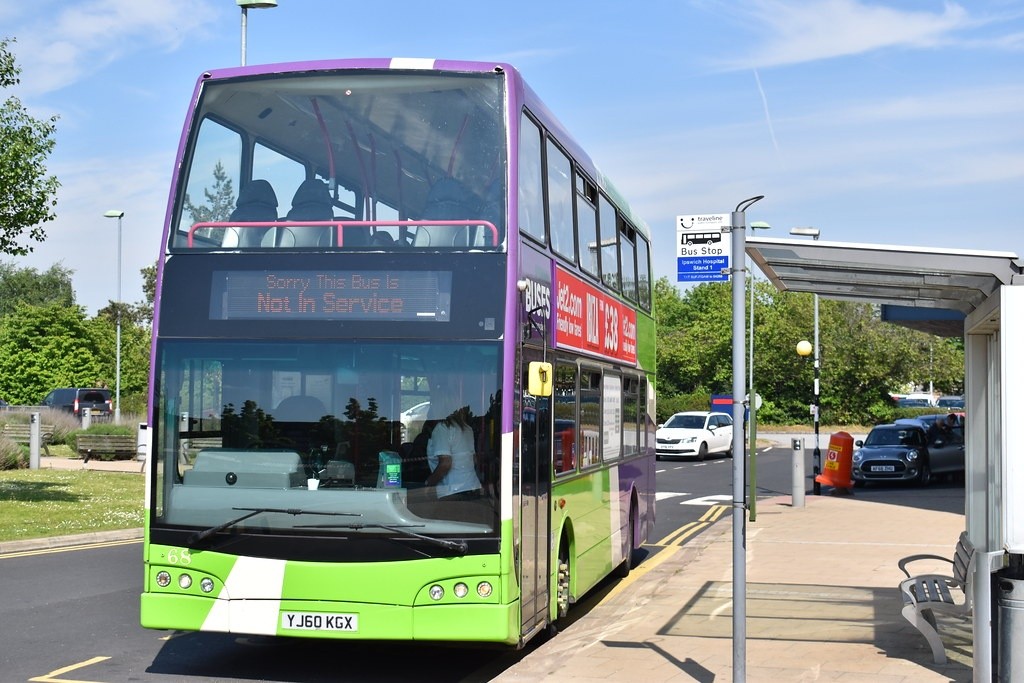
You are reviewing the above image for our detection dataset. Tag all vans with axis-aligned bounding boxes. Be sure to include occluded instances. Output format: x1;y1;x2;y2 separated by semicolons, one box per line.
39;388;114;426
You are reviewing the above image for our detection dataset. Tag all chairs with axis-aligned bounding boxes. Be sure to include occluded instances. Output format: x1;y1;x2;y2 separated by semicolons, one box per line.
223;178;497;255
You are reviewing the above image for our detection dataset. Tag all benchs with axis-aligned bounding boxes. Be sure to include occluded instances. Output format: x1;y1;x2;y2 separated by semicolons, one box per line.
78;433;146;464
2;424;55;456
897;532;974;664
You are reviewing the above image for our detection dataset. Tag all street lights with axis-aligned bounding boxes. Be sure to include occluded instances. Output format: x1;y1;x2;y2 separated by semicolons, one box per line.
100;210;124;424
789;226;822;496
748;221;771;526
233;0;278;66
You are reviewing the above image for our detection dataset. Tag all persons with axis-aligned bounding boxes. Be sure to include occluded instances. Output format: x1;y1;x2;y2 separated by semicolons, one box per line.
928;418;954;487
424;386;482;500
946;414;963;443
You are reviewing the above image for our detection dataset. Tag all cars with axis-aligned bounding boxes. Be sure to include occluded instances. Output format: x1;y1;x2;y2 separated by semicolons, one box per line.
888;392;965;409
138;58;657;650
895;414;966;484
852;424;932;486
400;401;432;444
654;412;735;462
